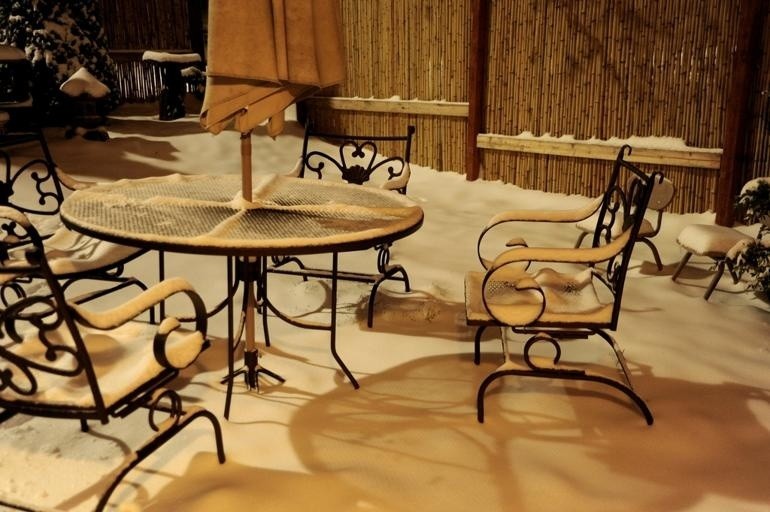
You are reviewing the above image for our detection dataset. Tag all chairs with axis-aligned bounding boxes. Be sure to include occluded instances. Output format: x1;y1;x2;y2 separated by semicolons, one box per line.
257;118;415;328
2;207;227;511
2;65;165;326
574;172;676;284
464;144;668;425
671;177;770;301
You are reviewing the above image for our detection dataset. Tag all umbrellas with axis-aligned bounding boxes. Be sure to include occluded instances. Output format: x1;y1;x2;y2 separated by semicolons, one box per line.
199;0;347;392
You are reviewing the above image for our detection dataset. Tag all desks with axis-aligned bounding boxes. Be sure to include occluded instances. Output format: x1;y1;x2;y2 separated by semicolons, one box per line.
60;173;425;421
